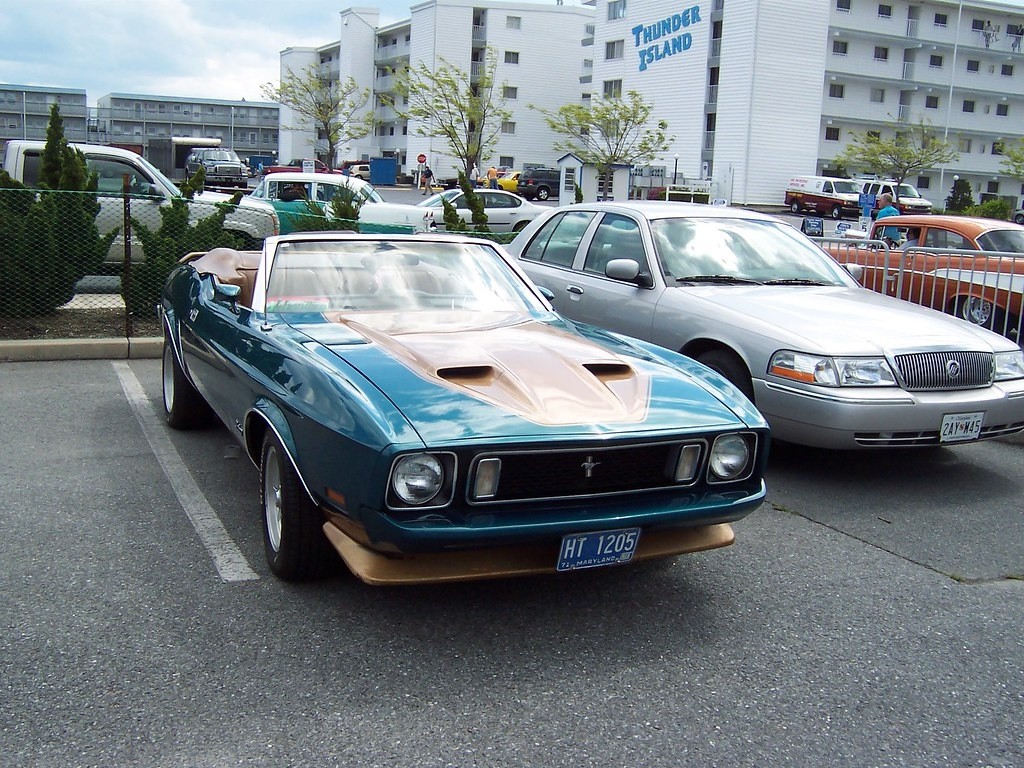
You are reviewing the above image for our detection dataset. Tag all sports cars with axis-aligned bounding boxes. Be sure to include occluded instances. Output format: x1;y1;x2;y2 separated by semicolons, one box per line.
156;228;771;586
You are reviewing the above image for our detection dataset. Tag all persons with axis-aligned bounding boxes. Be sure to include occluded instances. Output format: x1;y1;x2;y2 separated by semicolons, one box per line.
278;183;305;202
259;160;263;182
876;193;900;239
469;162;479;188
420;166;435;196
487;166;498;190
885;228;930;251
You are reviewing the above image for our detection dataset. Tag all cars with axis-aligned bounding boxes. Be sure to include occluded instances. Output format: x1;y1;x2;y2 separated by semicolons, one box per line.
503;198;1024;452
1011;210;1024;222
249;173;437;236
435;177;457;191
416;189;555;233
483;171;524;196
818;214;1024;332
456;166;509;189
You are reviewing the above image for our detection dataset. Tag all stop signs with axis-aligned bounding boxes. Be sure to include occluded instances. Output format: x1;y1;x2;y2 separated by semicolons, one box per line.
418;154;426;163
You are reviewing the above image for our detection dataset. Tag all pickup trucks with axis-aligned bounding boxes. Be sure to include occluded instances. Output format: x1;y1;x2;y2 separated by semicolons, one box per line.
263;158;354;192
0;140;280;272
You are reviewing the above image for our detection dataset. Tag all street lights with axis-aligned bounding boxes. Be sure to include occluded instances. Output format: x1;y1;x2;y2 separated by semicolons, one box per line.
673;153;680;189
953;175;959;210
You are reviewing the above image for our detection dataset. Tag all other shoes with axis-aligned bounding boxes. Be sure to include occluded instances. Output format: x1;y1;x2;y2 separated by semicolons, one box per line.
422;193;426;195
430;193;434;195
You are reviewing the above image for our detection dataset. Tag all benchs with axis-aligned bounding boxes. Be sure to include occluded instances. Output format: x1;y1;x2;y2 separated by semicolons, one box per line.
235;268;445;309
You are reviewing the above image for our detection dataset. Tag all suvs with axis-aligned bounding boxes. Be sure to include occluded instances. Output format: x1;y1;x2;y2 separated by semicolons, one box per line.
346;164;371;181
184;148;248;190
515;165;560;200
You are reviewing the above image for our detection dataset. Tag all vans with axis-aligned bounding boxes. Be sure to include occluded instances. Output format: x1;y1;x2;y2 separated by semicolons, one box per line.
784;175;865;220
853;179;933;215
